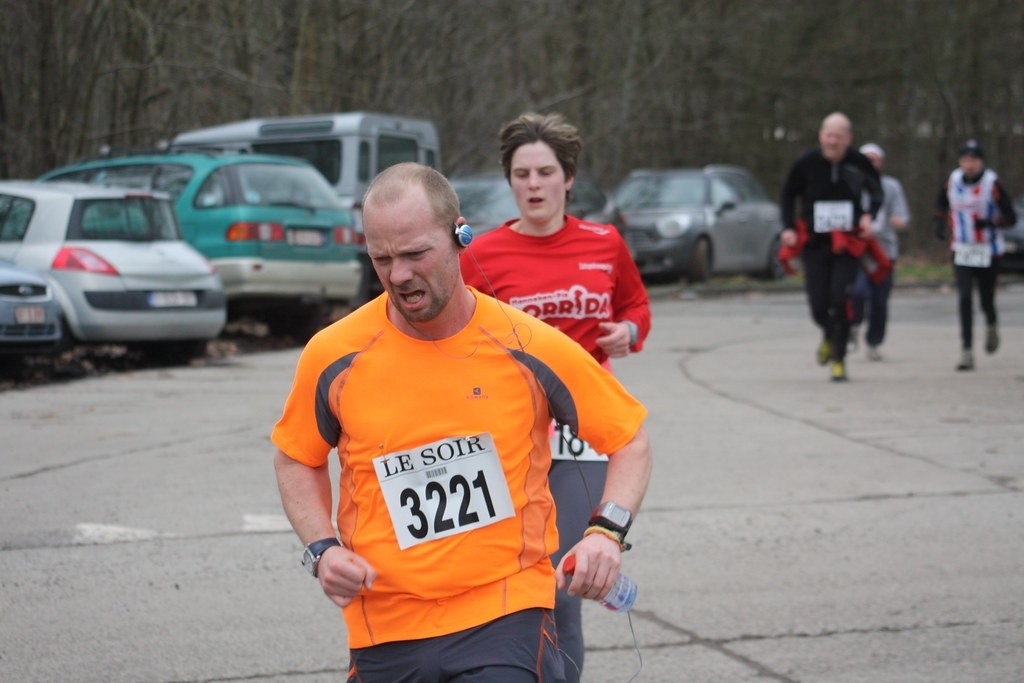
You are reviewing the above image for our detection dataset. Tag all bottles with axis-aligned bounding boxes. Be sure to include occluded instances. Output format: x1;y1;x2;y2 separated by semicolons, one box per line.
563;555;637;616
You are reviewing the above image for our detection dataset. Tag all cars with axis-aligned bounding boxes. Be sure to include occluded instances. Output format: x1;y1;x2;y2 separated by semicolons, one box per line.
0;151;362;344
448;169;628;243
0;179;228;367
989;197;1023;270
0;256;62;352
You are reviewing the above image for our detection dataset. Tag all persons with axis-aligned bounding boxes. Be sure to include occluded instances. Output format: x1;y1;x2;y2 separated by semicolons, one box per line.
460;111;651;683
781;111;885;380
848;143;909;363
269;162;653;683
934;139;1018;369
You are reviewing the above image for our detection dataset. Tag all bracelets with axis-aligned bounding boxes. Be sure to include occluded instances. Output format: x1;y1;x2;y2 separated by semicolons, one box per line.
621;320;637;346
584;525;631;552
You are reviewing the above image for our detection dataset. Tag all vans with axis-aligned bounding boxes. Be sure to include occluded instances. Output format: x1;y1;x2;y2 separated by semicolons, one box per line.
156;108;442;294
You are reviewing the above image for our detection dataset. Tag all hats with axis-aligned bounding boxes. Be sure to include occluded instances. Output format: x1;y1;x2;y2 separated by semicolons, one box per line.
957;139;983;159
858;142;885;161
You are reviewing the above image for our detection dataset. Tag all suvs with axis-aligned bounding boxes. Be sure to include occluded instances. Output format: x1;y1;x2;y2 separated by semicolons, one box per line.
581;163;790;285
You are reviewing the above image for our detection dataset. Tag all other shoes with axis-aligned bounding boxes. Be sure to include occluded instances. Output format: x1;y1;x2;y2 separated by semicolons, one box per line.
864;334;882;361
985;320;1000;353
829;359;845;380
817;336;832;365
846;324;858;352
956;349;973;371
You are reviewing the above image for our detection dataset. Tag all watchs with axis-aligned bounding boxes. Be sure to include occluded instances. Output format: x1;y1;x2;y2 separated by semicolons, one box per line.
301;537;342;578
587;501;633;536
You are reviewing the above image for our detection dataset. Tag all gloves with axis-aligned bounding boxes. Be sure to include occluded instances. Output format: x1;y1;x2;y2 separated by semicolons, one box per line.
934;216;946;240
974;217;994;231
